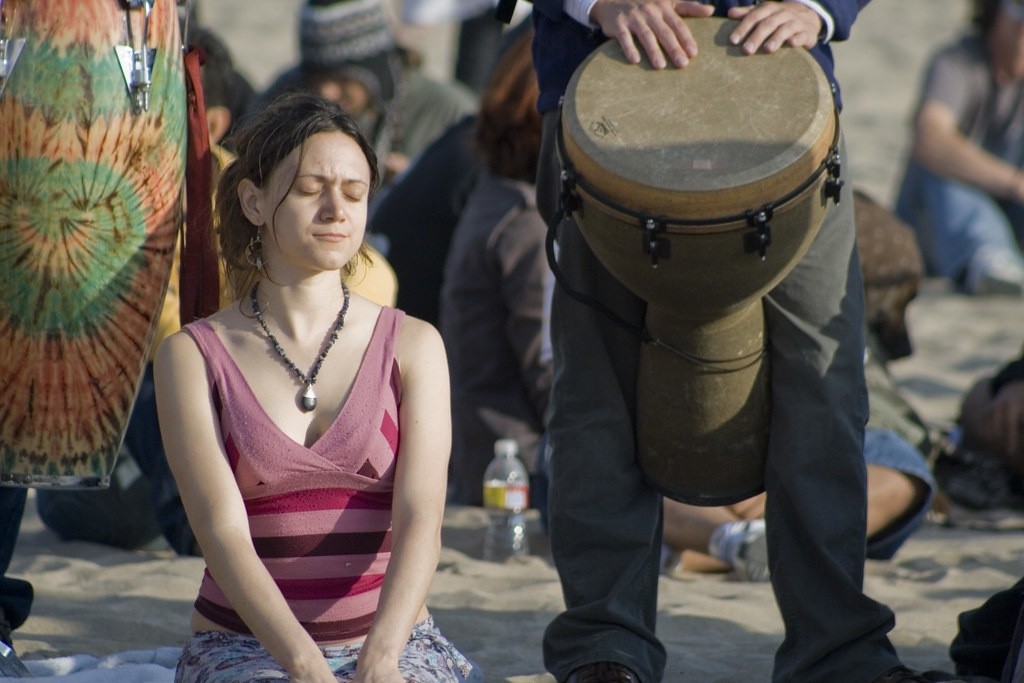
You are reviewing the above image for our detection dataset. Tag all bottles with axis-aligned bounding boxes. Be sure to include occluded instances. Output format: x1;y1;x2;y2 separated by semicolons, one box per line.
482;438;529;564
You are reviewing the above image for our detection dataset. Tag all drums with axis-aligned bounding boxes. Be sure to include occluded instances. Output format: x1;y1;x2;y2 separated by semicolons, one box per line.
545;12;843;508
1;0;189;492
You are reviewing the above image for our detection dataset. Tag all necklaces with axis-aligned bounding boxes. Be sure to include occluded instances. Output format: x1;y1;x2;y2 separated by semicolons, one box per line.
250;278;350;412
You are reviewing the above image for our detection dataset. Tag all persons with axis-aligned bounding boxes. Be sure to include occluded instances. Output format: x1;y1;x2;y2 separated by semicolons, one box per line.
0;485;34;635
35;0;938;583
893;0;1024;296
153;93;475;683
975;354;1024;510
532;0;927;683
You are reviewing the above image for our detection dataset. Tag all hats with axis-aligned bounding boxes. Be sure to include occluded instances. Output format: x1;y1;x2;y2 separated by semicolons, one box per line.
297;0;400;113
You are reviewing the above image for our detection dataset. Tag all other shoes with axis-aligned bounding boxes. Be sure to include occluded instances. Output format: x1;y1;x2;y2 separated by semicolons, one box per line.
967;245;1024;297
708;519;771;581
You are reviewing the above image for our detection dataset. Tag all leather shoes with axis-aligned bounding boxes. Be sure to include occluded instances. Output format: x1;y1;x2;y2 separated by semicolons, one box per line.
563;661;641;683
872;665;966;683
